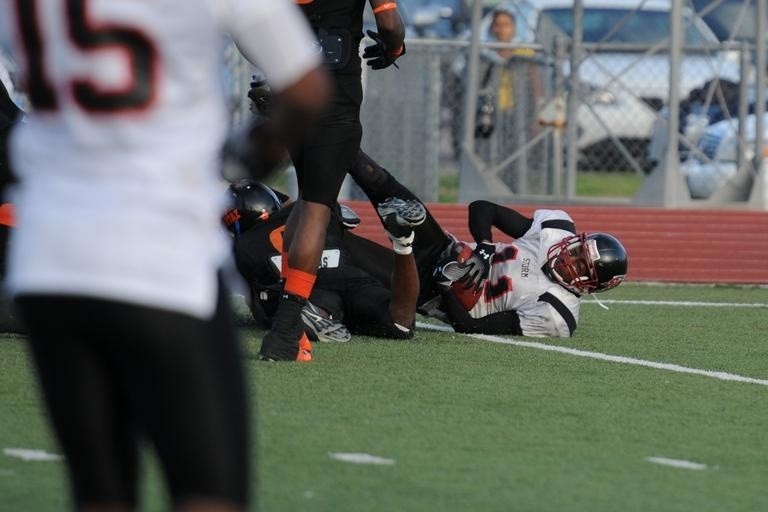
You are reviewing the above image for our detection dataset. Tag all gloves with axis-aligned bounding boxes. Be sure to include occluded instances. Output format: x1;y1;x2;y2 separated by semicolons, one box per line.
459;242;495;297
431;240;473;294
362;29;405;69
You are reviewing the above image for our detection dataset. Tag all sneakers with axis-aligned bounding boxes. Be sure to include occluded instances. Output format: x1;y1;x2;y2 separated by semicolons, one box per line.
339;204;360;231
297;326;314;354
258;341;313;363
247;86;270;109
378;198;426;248
299;301;352;343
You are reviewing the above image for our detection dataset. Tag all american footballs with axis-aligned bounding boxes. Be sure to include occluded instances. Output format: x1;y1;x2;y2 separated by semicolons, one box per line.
450;242;482;310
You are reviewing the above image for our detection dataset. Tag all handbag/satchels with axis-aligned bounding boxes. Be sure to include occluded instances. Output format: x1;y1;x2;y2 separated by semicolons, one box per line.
473;93;495;139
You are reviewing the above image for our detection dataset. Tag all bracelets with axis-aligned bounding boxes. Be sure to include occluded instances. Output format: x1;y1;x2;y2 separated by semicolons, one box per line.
373;3;397;14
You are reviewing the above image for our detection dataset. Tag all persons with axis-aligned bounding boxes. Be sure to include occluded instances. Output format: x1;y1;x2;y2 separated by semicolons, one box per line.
253;0;405;362
490;11;542;106
3;0;326;512
231;148;629;339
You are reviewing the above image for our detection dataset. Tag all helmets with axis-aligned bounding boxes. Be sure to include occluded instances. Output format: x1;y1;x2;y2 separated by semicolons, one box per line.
546;232;627;295
223;180;282;230
